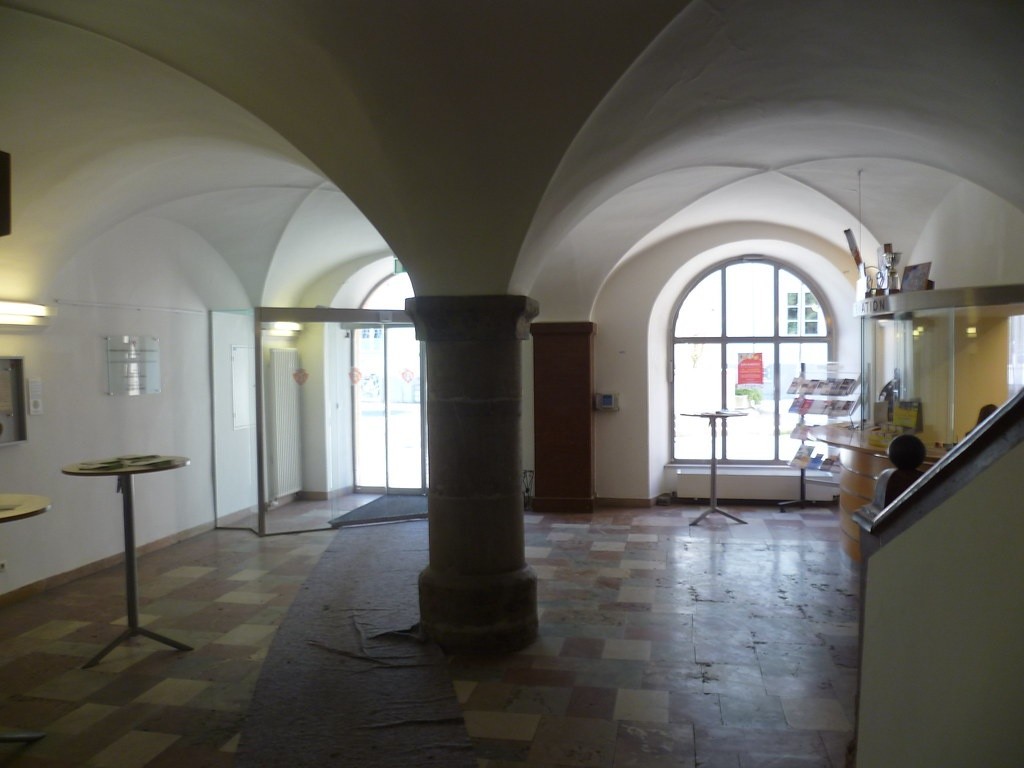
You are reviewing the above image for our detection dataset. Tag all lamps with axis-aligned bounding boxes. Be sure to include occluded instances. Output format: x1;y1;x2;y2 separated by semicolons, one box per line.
0;301;58;328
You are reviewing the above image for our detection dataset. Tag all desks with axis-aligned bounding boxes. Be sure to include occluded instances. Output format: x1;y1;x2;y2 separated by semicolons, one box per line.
62;455;195;668
0;491;54;743
681;413;748;525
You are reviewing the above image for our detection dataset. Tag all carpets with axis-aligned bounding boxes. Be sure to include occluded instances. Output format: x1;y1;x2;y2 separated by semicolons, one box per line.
237;490;477;768
